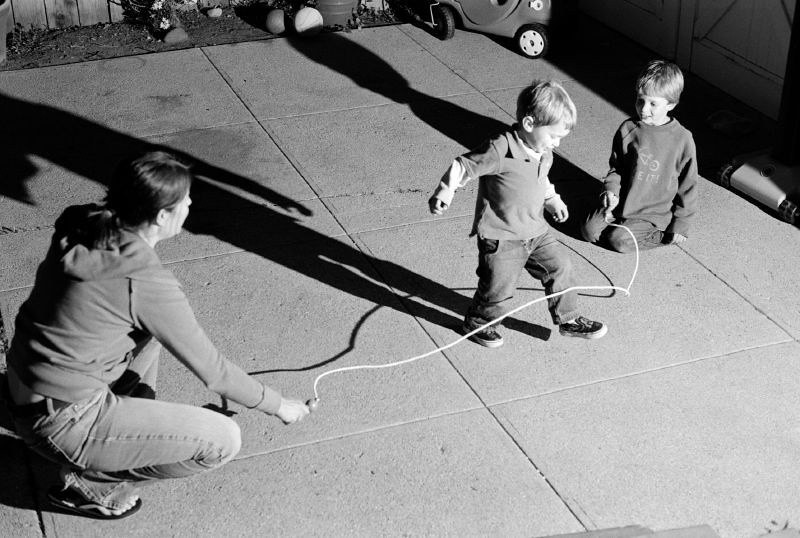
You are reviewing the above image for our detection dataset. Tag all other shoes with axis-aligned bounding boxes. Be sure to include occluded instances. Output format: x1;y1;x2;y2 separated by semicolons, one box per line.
559;316;607;340
464;316;503;347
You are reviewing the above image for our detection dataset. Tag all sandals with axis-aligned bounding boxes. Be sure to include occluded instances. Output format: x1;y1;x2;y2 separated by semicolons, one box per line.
48;485;142;519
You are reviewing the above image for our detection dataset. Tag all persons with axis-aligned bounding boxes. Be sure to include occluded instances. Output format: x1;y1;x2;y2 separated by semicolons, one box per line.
427;79;608;347
579;59;698;255
4;151;309;519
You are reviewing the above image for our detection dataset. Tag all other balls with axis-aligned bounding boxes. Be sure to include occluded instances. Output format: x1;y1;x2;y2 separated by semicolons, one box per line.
294;7;325;37
266;9;286;34
